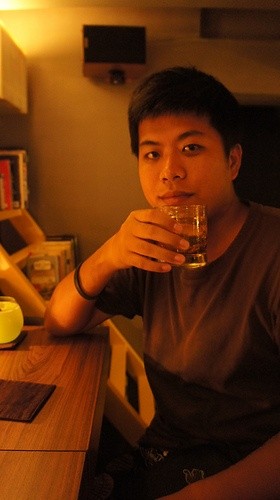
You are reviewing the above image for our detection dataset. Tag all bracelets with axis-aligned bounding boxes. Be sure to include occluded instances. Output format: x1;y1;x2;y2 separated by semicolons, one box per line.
75;259;106;301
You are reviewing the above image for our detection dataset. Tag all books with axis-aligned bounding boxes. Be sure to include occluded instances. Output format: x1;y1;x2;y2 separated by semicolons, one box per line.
0;150;29;212
26;239;77;301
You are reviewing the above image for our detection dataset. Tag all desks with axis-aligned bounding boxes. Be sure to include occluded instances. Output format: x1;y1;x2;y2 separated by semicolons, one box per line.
0;326;110;500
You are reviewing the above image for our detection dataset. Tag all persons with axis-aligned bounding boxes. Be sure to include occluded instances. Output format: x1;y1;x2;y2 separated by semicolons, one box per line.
44;65;280;500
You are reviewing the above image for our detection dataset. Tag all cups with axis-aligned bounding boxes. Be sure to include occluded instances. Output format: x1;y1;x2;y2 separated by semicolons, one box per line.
0;296;23;343
155;205;208;269
